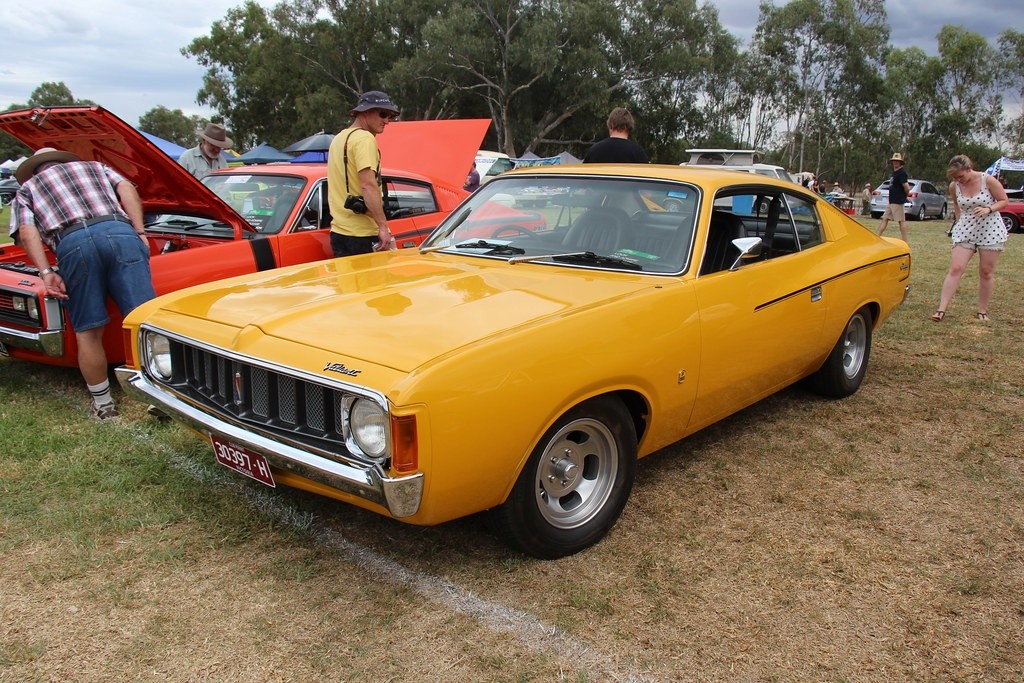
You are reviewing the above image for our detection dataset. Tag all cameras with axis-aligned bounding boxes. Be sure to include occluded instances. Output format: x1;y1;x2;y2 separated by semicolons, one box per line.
344;195;368;215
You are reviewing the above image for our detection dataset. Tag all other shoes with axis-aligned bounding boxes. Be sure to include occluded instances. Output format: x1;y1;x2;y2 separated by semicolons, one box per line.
91;401;120;420
147;404;167;417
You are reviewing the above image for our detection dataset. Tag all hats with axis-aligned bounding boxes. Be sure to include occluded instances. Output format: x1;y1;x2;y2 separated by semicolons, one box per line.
350;91;400;117
890;153;906;165
15;148;81;187
833;182;839;185
864;183;872;188
198;123;233;148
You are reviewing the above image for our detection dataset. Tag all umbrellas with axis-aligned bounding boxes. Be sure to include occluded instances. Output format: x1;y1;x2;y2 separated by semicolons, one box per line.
288;151;329;162
278;129;336;162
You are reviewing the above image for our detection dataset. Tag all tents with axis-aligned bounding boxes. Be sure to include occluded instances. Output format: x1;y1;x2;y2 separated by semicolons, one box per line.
518;151;541;159
0;155;29;174
556;150;582;165
138;130;188;162
226;145;295;162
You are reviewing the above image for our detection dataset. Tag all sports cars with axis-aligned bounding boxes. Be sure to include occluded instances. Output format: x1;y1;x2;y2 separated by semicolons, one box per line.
0;103;548;371
113;162;914;561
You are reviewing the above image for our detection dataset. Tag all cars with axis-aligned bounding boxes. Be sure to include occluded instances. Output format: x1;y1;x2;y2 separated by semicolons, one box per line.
869;178;949;221
999;198;1024;234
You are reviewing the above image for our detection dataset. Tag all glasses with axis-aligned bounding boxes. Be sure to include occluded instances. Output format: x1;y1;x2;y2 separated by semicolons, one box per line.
373;109;393;121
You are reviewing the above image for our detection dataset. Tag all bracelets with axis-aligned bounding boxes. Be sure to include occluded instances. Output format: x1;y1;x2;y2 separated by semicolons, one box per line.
136;232;144;235
988;207;993;213
38;266;55;277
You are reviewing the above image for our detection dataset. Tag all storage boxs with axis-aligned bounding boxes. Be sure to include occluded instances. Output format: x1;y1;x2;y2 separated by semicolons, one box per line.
843;209;855;215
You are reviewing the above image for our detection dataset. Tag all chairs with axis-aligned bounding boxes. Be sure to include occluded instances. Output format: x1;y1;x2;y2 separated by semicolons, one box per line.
665;211;748;275
263;193;296;233
561;207;633;253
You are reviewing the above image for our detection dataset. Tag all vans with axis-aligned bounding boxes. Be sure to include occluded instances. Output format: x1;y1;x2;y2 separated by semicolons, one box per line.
659;148;808;215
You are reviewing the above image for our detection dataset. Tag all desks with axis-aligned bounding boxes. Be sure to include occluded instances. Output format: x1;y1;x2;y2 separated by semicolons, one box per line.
825;197;856;210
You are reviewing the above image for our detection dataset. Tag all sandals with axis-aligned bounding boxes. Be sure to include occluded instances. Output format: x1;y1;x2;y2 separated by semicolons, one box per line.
930;310;945;321
977;313;988;320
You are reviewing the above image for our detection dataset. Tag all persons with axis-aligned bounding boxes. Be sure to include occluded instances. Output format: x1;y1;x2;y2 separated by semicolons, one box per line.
999;174;1009;188
583;107;652;217
9;147;173;424
832;182;843;208
802;176;826;195
861;183;872;215
464;161;480;193
176;123;242;214
328;91;400;257
929;154;1010;321
876;152;911;248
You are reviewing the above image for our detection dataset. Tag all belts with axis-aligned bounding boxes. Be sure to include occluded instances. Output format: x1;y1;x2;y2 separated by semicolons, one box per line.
55;215;132;246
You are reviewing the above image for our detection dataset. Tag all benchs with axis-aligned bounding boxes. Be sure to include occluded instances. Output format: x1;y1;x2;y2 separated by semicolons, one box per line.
630;210;815;264
381;200;433;215
